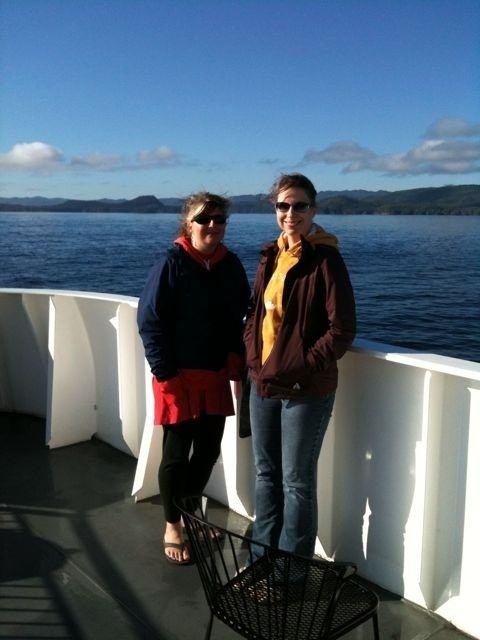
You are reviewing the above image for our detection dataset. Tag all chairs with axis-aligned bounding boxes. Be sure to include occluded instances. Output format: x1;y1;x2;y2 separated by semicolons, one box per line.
170;488;383;638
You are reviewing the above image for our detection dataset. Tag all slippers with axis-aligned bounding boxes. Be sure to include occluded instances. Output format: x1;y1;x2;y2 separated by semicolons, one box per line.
162;521;221;566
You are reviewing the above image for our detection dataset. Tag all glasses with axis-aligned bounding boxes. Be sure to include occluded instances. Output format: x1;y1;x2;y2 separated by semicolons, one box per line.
191;212;229;225
275;201;314;214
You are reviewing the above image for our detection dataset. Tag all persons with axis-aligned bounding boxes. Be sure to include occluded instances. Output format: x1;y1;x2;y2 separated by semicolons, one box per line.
233;173;357;609
136;187;251;565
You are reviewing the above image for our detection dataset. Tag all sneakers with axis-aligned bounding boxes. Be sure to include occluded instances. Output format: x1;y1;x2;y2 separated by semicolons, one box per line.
228;565;302;603
